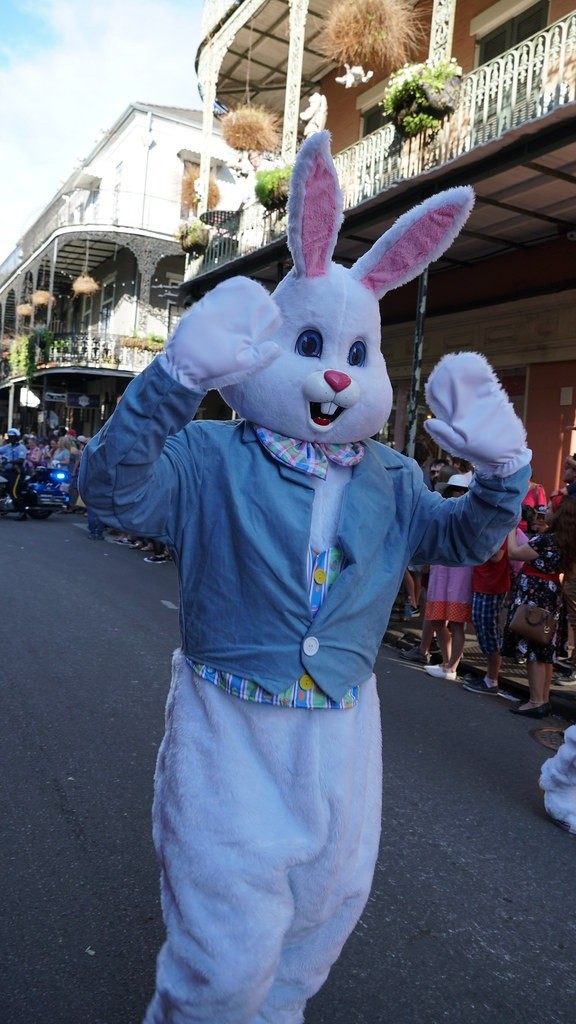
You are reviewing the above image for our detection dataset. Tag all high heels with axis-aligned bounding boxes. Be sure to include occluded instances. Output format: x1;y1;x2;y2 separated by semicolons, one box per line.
519;701;551;718
509;704;544;720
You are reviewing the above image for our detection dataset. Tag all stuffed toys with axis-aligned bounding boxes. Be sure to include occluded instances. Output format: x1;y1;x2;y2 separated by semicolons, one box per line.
78;129;532;1024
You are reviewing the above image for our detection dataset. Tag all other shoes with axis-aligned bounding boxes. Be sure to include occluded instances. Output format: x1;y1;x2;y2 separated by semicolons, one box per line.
426;668;456;680
400;649;430;664
89;529;172;563
557;657;574;668
390;605;421;620
555;671;576;685
423;664;439;669
414;637;441;653
14;512;27;520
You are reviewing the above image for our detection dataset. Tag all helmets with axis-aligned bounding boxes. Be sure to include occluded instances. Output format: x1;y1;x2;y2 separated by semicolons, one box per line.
8;428;21;441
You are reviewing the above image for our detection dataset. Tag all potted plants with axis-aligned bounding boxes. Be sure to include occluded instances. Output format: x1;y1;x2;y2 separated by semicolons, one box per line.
175;223;209;252
255;166;293;211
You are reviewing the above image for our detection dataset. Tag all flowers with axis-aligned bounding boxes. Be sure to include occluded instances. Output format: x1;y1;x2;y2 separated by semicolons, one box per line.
379;57;463;137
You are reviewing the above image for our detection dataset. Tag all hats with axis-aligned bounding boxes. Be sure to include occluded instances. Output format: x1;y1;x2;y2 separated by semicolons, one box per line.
68;429;77;436
444;475;470;488
533;505;546;515
438;465;458;481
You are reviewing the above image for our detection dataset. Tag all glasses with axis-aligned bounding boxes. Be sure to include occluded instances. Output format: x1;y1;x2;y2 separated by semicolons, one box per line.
451;486;468;493
430;470;439;475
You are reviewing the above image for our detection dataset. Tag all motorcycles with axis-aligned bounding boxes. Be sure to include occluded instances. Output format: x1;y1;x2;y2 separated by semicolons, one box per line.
0;454;72;522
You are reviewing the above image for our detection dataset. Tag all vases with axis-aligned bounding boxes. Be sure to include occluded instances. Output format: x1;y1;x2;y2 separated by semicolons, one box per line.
391;76;461;135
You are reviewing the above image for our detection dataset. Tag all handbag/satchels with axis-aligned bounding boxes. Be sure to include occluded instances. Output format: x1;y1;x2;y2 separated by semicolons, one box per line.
509;604;556;645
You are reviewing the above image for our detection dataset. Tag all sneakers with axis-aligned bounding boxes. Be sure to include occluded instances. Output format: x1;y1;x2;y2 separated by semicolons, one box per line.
464;678;483;684
462;681;498;696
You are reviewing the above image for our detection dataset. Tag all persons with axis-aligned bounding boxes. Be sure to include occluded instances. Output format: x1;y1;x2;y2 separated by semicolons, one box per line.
86;508;166;564
246;150;272;175
398;442;576;719
334;63;373;89
300;92;321;139
0;427;86;524
